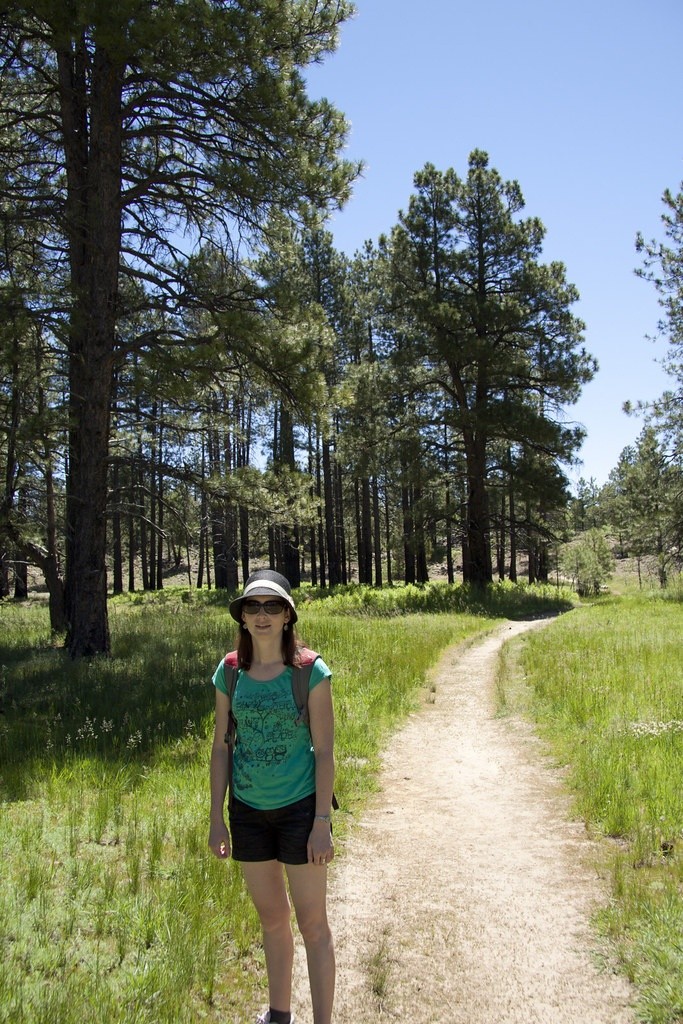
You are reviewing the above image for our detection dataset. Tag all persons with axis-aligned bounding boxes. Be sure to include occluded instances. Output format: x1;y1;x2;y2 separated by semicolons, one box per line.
208;570;338;1024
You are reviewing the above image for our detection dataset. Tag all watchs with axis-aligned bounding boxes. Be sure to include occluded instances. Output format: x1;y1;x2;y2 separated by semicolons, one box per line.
316;816;332;823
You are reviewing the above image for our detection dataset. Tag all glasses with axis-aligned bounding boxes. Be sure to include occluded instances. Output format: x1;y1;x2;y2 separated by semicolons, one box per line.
241;599;285;614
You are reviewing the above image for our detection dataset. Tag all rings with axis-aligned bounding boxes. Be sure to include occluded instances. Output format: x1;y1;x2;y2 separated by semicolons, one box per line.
321;859;326;863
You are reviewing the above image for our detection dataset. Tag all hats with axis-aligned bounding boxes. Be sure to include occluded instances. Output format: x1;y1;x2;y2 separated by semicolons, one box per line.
229;570;298;623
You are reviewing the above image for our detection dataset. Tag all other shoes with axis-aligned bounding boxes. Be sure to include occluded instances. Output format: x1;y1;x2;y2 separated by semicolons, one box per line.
255;1010;295;1024
